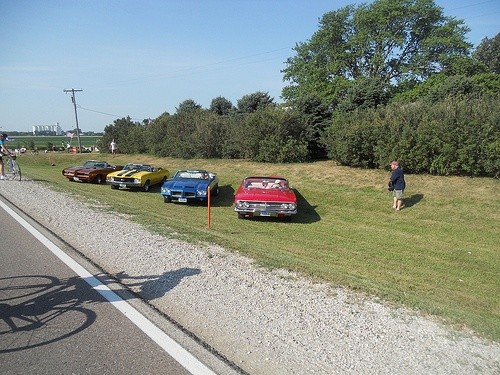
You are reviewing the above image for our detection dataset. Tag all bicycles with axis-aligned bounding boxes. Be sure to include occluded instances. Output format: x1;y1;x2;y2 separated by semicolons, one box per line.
2;152;22;181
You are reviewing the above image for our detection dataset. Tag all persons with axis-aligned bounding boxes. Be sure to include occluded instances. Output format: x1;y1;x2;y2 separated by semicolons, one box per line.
67;141;70;149
109;138;118;158
0;133;14;180
62;141;66;150
200;172;205;179
389;161;405;210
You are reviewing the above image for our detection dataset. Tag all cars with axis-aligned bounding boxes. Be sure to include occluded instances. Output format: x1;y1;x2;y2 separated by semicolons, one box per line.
160;169;219;204
105;162;170;192
233;175;298;222
61;159;123;184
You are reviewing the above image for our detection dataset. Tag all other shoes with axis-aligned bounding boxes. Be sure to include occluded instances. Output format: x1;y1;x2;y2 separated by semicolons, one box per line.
0;175;8;179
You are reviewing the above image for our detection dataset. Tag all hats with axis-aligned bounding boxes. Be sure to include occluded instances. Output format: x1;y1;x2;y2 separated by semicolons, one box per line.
389;160;399;165
